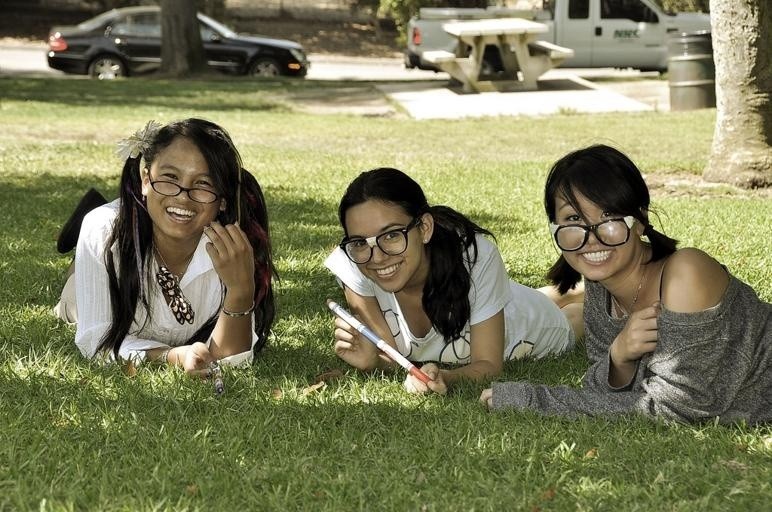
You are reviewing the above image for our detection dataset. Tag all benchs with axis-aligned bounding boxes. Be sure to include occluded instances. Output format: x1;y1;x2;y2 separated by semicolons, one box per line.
529;41;574;92
422;50;469;94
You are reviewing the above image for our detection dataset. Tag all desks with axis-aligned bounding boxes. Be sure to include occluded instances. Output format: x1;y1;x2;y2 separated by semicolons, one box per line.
442;18;549;94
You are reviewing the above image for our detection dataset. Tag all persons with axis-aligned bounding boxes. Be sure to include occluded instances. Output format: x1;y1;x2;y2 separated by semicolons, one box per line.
51;116;280;380
478;144;771;428
322;167;587;394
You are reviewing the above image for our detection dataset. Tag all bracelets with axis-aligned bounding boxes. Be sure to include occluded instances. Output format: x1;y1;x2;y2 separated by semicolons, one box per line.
221;299;256;318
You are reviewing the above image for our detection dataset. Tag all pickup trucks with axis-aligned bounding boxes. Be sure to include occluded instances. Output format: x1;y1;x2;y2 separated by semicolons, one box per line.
402;1;710;82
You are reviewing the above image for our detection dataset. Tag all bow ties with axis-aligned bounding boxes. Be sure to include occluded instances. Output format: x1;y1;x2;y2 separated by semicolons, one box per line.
157;267;195;324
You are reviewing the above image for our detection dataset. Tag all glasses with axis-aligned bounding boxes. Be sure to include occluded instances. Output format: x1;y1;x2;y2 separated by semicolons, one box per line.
146;166;222;205
339;218;417;265
548;206;642;252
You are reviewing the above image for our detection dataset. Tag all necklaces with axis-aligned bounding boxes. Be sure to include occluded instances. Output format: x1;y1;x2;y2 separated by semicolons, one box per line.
612;263;646;317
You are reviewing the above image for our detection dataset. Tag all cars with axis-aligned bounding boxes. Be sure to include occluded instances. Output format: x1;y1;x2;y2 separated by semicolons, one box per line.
47;5;309;79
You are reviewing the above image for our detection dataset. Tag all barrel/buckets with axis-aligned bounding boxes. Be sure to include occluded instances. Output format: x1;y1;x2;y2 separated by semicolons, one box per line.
666;31;716;112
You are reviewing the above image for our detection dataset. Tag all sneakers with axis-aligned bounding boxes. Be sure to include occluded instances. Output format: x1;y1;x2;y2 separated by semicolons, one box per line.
55;187;108;254
